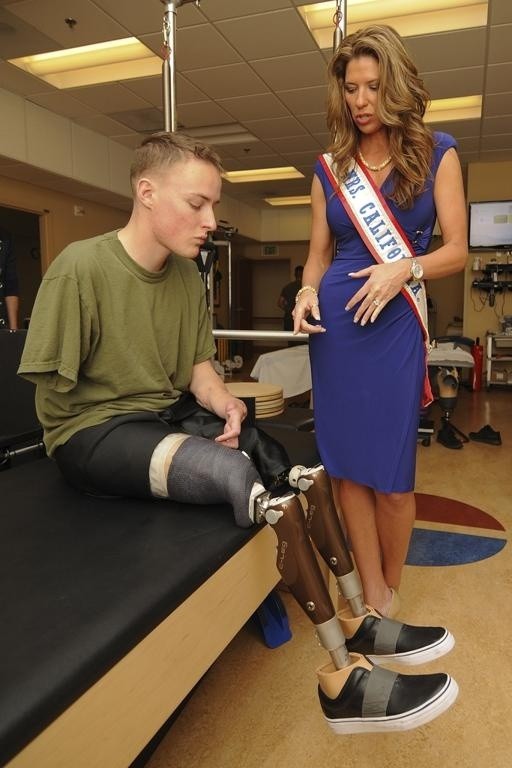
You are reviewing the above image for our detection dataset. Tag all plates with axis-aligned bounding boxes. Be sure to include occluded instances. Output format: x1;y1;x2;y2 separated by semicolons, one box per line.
222;379;285;423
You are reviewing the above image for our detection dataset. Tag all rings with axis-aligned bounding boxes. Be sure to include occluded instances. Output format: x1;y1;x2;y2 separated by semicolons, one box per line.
311;304;320;307
373;300;379;305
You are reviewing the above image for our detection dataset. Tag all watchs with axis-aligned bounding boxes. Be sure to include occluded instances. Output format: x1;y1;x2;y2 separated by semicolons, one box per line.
410;256;424;285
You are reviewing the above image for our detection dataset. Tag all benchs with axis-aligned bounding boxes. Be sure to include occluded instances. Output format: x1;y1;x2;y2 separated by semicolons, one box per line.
0;326;327;767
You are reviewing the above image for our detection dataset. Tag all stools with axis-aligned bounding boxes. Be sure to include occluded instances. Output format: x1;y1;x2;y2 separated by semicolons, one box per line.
256;405;317;436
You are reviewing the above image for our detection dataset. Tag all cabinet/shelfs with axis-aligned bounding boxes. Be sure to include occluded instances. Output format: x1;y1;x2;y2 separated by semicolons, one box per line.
482;330;511;390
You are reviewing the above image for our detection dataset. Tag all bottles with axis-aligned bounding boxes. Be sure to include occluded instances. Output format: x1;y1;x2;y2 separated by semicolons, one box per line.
471;335;484;391
471;256;481;271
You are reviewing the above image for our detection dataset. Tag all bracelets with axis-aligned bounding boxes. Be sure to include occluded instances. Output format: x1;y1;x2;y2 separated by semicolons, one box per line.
295;286;318;302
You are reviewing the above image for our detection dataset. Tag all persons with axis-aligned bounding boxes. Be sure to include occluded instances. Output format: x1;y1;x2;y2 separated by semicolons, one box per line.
15;133;460;735
1;268;19;331
275;265;304;348
292;23;468;621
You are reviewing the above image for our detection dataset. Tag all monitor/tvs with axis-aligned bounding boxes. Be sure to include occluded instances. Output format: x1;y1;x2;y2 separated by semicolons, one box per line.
468;200;512;251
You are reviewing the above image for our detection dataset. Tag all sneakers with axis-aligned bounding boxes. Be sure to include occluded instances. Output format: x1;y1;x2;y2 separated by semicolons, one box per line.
333;604;456;667
312;669;461;739
468;424;503;446
436;428;465;449
